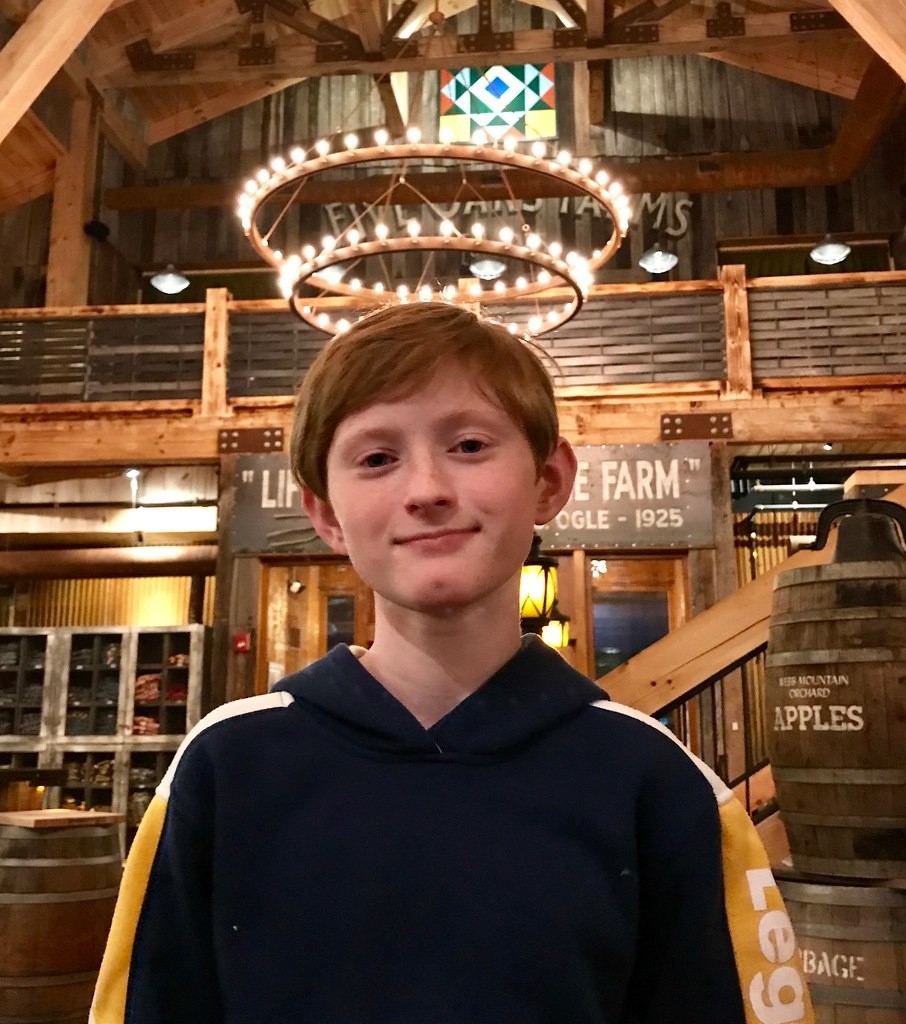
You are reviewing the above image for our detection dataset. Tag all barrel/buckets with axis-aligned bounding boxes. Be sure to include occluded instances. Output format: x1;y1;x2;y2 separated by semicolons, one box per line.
766;566;906;879
0;823;123;1024
780;876;906;1024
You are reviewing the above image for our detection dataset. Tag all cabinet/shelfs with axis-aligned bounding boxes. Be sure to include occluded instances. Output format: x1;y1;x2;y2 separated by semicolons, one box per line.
0;623;207;870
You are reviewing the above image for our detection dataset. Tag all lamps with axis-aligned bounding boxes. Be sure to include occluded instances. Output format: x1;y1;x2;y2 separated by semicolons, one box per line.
519;530;560;638
150;263;190;295
234;0;635;344
541;597;577;651
809;233;851;265
637;241;679;273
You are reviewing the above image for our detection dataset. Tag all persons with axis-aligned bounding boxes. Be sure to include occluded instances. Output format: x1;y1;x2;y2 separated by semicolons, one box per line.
89;301;816;1024
